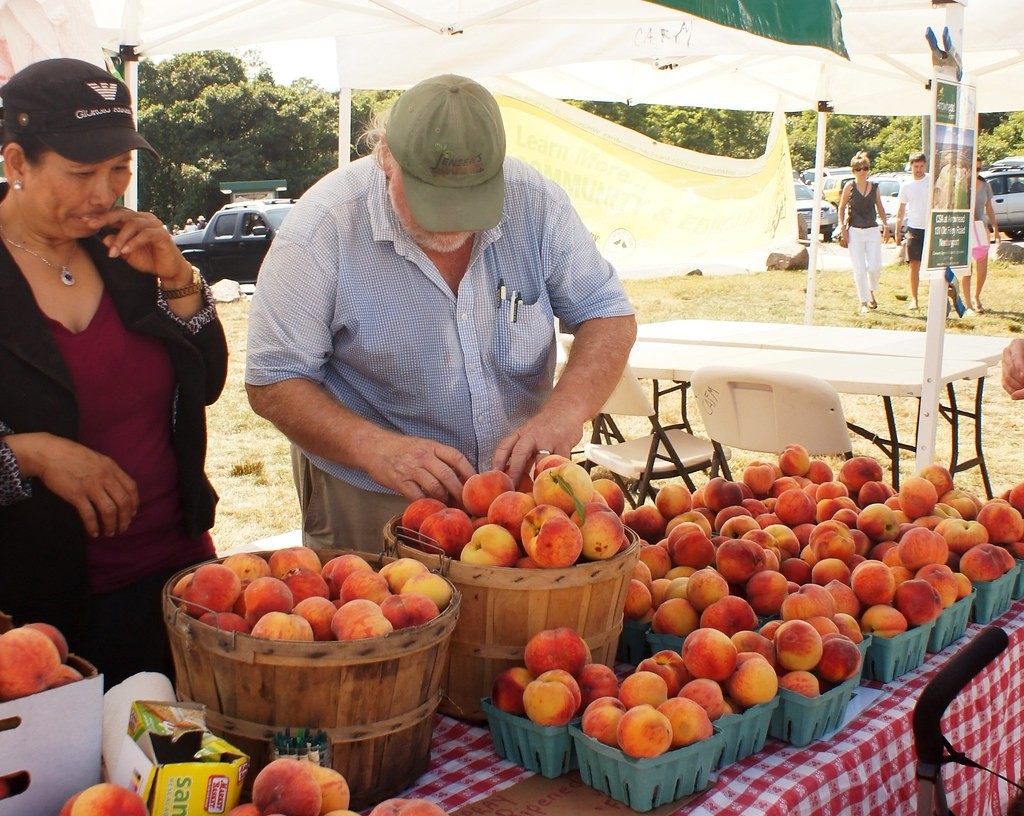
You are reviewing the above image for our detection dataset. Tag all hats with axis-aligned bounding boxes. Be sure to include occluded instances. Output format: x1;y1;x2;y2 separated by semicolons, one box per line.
0;58;162;165
386;75;505;228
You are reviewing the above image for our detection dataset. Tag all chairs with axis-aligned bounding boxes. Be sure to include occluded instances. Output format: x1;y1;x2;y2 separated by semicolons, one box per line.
561;339;855;515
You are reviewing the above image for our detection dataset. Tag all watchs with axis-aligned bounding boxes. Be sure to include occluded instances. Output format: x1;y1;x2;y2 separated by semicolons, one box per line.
162;266;201;299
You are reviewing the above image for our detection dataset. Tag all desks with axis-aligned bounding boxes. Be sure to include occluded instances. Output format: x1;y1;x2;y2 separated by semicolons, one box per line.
358;600;1024;815
553;321;1016;501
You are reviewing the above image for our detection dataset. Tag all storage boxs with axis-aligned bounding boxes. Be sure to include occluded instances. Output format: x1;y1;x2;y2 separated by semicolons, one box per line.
0;554;1024;816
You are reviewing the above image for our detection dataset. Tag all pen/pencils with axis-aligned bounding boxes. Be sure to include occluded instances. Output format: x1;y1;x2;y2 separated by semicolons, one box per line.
274;727;327;766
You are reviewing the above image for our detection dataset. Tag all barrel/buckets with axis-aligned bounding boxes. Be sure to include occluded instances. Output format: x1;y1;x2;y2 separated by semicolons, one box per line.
161;544;462;809
380;506;642;722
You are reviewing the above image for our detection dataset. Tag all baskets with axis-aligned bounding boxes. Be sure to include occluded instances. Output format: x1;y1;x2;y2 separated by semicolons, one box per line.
479;531;1024;811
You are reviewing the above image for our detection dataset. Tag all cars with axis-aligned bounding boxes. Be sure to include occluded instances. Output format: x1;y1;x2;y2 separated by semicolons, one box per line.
170;199;299;285
811;175;856;208
978;157;1024;240
794;179;837;234
867;172;912;241
802;167;854;184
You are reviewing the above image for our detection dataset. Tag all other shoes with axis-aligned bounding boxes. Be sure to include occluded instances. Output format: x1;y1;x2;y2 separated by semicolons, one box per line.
866;292;876;310
861;305;869;313
974;298;984;313
910;298;919;310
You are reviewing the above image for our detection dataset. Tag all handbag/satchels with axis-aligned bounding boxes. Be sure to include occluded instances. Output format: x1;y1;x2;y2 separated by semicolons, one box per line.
840;229;849;248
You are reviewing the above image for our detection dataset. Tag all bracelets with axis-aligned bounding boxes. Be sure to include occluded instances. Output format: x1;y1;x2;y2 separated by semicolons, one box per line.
883;225;888;227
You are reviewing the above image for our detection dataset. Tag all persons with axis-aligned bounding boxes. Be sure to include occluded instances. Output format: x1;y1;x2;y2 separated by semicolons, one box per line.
895;152;930;310
839;151;890;313
1001;338;1024;401
173;216;207;235
961;157;1001;313
245;74;637;555
0;58;230;694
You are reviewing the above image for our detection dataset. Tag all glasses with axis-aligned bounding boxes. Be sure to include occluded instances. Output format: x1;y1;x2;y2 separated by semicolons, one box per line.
855;166;869;172
977;168;981;171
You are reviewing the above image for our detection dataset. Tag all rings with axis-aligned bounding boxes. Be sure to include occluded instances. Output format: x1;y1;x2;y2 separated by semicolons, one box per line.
537;450;551;454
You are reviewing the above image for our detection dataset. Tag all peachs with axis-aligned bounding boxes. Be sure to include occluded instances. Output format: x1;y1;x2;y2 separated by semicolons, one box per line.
227;758;361;816
491;443;1024;758
171;546;453;640
368;798;449;816
58;783;150;816
0;624;84;799
402;454;630;567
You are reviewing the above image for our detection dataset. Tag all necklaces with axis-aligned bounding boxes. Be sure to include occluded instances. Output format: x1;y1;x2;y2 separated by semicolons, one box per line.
0;226;77;286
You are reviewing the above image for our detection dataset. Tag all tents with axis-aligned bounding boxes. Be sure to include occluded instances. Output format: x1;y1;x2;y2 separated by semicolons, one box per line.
0;0;1024;472
